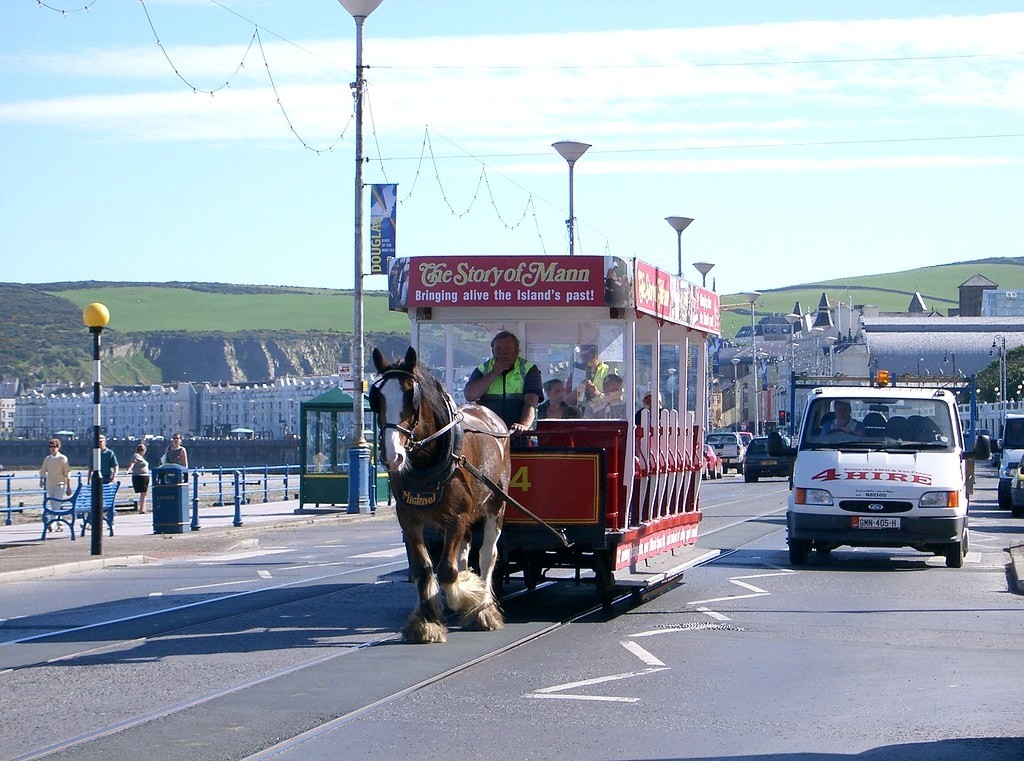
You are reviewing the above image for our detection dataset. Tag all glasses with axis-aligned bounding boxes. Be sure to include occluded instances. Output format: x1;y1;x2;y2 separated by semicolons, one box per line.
172;437;180;440
50;446;57;448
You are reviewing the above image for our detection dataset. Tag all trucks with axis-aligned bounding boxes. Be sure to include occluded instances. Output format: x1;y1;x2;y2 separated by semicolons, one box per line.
767;369;991;567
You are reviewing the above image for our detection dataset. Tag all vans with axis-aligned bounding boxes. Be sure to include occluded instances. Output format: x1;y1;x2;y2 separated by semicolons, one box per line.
992;413;1024;518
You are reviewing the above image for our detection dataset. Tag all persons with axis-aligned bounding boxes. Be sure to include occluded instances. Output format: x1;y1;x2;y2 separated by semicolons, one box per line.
310;452;328;472
820;400;865;436
80;435;118;530
584;374;623;419
125;444;151;514
636;390;663;424
165;433;188;468
565;345;618;412
464;332;544;448
40;439;72;532
538;379;583;419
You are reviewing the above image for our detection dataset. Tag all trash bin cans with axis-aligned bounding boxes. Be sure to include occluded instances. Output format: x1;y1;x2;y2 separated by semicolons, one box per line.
150;463;190;533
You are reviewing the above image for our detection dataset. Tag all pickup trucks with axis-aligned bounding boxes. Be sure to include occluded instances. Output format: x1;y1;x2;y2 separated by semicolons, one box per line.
704;431;754;477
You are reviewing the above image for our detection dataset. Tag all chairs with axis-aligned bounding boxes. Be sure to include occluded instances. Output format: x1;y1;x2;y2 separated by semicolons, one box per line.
817;411;943;441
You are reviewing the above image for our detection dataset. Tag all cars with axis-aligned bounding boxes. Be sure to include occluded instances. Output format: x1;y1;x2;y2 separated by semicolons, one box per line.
743;436;795;483
702;443;723;480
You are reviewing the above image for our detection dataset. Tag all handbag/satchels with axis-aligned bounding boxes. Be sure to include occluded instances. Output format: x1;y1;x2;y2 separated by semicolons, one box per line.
161;446;170;464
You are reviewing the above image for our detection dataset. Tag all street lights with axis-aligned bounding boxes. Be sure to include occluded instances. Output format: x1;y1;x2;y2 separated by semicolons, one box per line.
551;141;593;257
992;334;1007;419
810;327;825;375
943;350;955;376
81;303;110;555
784;313;802;446
337;0;385;514
730;359;741;431
743;291;767;433
664;216;695;276
918;357;924;375
691;262;715;288
824;336;837;376
990;344;1002;425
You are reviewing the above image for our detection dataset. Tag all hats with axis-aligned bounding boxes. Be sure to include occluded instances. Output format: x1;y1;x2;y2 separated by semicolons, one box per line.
642;391;662;403
99;434;106;441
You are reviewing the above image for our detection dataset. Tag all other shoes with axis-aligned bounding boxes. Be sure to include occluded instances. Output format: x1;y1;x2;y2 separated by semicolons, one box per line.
55;529;63;532
47;525;52;532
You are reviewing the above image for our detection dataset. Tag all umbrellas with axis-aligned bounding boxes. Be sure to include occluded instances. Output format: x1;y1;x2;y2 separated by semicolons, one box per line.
229;428;254;434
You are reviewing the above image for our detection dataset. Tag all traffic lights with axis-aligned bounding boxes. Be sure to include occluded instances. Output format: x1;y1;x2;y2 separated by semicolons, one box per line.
778;410;786;425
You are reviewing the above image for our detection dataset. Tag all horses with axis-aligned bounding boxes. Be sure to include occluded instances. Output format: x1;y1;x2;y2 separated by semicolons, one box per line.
367;345;513;644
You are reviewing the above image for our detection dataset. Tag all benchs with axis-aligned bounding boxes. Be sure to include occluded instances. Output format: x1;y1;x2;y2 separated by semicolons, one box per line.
41;481;121;541
524;421;630;488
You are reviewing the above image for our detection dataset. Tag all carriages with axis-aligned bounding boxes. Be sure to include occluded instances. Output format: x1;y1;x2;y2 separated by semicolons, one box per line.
367;254;720;644
